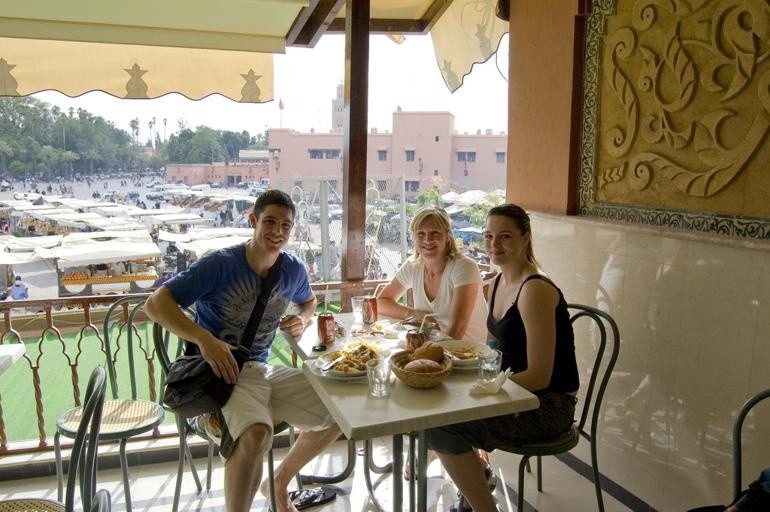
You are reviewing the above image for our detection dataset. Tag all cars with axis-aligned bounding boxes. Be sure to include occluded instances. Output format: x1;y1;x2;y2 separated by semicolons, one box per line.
157;178;268;197
52;169;161;188
299;192;343;223
103;191;117;198
146;192;163;200
373;198;395;208
126;190;139;198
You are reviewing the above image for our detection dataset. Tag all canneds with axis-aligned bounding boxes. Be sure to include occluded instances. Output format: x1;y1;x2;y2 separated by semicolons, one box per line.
318;313;334;345
406;330;425;350
363;295;377;323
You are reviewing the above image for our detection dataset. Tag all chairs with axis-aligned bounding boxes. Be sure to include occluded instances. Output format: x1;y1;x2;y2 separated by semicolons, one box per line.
84;487;113;512
730;389;769;512
497;294;626;511
144;297;312;511
54;288;189;512
0;359;110;512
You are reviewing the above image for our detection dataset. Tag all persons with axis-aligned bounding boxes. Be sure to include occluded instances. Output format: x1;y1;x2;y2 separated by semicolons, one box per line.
144;190;343;512
211;208;250;227
376;205;491;478
12;275;28;300
35;182;161;209
108;260;176;288
409;204;579;511
592;228;669;426
291;220;387;280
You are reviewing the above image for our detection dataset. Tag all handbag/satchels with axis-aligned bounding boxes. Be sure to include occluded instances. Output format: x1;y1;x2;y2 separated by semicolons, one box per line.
159;341;250;419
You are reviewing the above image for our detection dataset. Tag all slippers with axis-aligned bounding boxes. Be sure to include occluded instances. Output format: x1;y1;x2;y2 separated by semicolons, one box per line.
287;484;338;511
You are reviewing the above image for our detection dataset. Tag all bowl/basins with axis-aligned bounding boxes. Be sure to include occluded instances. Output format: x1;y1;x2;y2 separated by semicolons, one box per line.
387;349;454;390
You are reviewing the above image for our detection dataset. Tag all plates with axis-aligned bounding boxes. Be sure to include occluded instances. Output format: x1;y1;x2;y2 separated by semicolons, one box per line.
309;350;376;381
370;320;437;333
338;336;387;348
432;339;494;373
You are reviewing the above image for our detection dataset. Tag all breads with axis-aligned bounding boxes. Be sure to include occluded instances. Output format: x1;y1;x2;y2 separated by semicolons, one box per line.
410;342;443;362
404;359;443;373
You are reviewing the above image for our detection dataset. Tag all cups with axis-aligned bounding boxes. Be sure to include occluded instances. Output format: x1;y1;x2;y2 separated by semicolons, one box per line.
477;347;503;384
365;359;392;398
350;294;365;323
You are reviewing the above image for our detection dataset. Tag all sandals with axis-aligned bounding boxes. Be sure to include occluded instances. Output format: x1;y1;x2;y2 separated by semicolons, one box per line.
448;464;498;511
